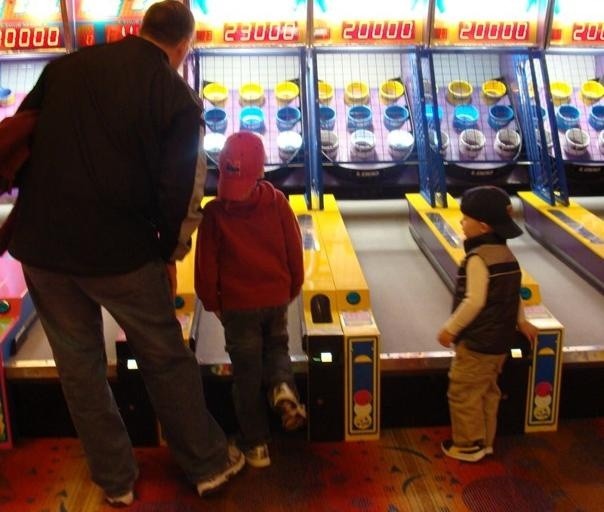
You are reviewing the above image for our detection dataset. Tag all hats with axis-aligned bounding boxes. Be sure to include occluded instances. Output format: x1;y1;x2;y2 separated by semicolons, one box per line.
215;131;266;200
460;186;523;239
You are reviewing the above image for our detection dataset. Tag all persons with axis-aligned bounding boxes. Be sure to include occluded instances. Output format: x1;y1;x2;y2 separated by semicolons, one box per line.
437;186;523;464
6;0;245;507
195;131;307;469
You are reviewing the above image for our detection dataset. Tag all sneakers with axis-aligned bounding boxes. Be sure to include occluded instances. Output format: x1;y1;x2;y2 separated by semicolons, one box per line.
106;491;135;505
273;382;307;433
486;445;494;458
230;438;271;467
440;439;487;463
196;444;246;497
